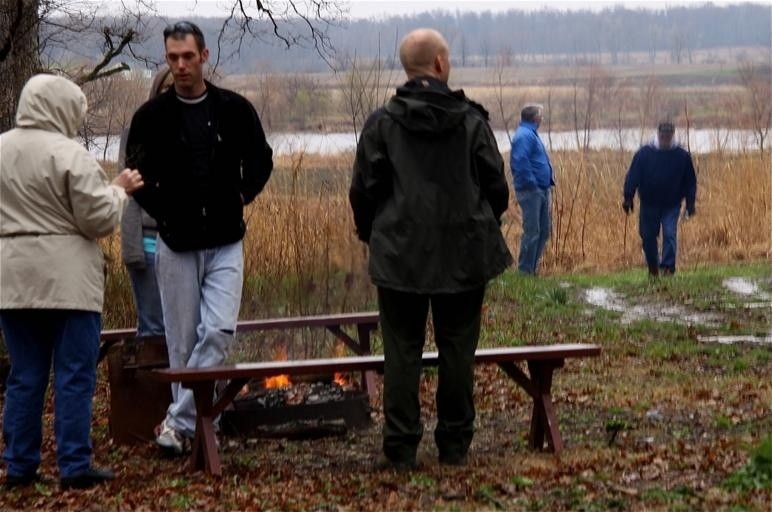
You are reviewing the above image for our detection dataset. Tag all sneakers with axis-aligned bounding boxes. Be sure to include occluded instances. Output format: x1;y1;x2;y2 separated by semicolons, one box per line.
439;448;472;465
153;419;220;453
389;445;416;468
649;262;673;277
60;468;115;490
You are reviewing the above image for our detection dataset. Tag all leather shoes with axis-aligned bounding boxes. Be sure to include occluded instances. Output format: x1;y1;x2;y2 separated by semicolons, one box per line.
5;467;42;486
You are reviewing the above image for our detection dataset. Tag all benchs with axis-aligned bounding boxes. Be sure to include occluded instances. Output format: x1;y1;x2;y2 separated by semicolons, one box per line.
94;310;383;439
150;344;602;478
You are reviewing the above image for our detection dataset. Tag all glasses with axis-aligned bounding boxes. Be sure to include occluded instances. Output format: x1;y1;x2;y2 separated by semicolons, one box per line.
163;22;195;35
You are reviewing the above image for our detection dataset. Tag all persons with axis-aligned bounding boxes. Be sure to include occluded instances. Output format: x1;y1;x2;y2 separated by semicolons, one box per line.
623;121;697;280
349;27;513;472
0;73;144;491
509;105;554;274
117;66;180;338
124;21;274;465
